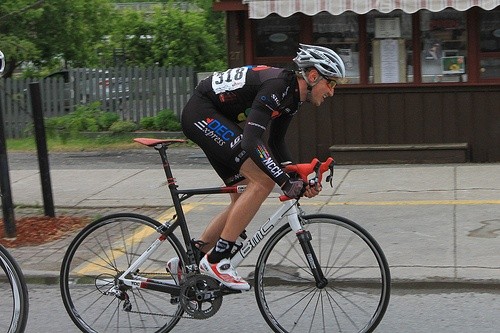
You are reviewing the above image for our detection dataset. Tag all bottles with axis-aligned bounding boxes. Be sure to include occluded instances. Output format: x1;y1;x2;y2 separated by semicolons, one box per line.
230;228;247;257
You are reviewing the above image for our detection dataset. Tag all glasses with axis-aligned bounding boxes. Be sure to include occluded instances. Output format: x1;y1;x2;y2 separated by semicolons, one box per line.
314;66;338;91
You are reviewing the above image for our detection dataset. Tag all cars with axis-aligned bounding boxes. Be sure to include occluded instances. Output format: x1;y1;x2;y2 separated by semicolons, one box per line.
13;69;130;111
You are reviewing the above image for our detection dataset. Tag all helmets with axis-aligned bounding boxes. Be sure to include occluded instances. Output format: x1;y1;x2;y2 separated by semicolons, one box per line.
292;42;345;80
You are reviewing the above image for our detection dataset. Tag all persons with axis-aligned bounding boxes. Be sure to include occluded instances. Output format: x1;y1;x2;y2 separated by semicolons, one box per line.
165;43;346;291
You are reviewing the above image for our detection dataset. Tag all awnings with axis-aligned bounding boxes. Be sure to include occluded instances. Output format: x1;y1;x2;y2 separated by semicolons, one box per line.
243;0;500;20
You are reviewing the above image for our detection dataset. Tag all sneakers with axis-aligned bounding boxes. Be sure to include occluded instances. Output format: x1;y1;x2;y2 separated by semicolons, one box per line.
198;245;250;290
166;257;186;286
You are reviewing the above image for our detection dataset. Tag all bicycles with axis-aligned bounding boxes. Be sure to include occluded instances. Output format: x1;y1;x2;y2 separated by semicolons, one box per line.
0;244;29;333
59;138;391;333
